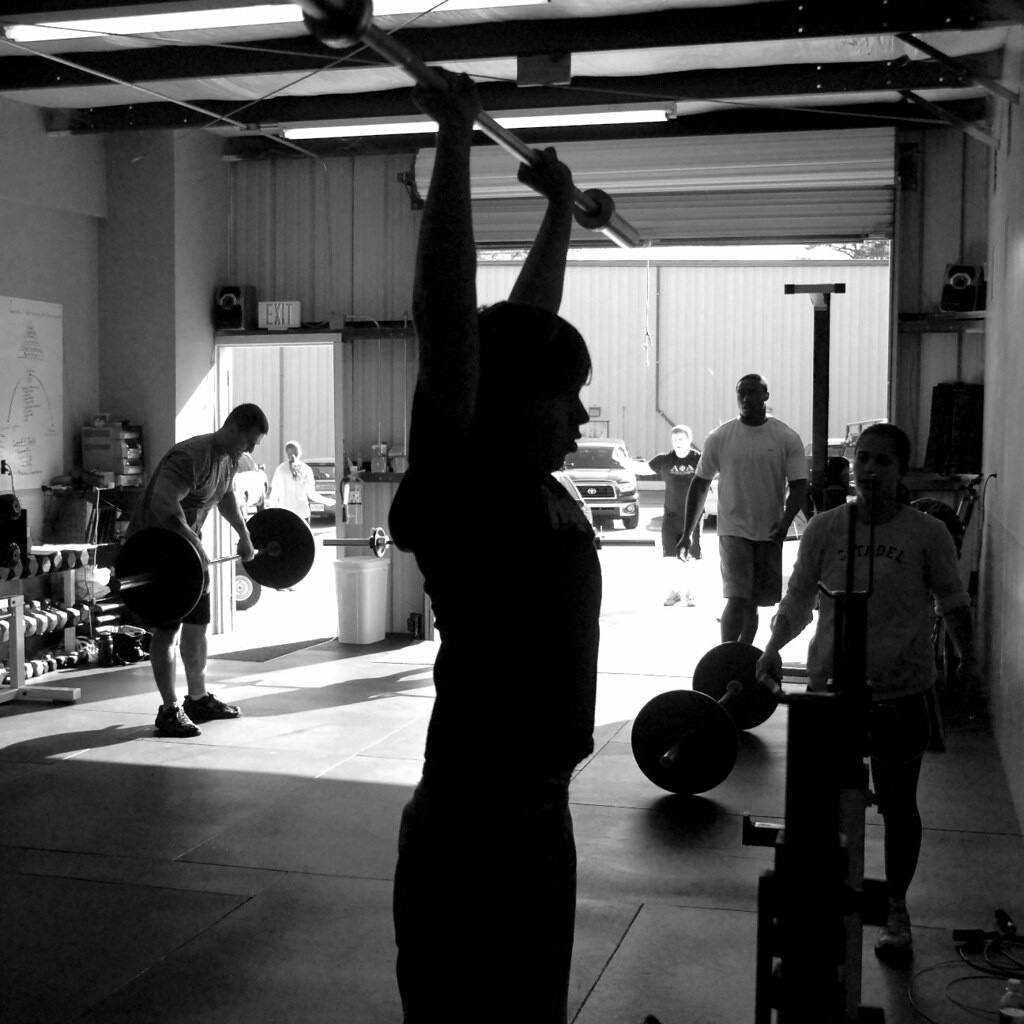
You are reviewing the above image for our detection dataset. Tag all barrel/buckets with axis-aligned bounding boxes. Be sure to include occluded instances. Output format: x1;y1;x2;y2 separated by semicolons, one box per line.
333;556;390;645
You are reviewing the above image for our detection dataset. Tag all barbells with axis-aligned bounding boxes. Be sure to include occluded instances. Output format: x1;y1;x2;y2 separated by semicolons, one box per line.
323;527;657;558
296;2;646;250
629;639;783;797
110;505;324;624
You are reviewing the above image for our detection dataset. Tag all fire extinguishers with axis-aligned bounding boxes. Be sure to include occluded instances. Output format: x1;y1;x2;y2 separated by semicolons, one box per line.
339;465;366;527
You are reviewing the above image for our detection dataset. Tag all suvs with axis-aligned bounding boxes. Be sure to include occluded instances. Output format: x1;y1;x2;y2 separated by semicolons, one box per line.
782;437;846;540
563;438;645;529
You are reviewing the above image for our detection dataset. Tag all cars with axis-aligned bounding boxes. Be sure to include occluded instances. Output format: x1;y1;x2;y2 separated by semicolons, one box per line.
232;452;269;517
552;471;593;525
302;455;336;520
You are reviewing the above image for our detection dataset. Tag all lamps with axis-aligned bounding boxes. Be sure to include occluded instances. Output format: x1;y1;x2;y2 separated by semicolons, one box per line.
589;407;600;418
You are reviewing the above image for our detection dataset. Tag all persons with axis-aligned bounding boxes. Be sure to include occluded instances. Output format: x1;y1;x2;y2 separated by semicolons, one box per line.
648;425;705;608
124;403;269;738
388;65;602;1024
266;440;337;592
677;374;810;645
757;424;978;958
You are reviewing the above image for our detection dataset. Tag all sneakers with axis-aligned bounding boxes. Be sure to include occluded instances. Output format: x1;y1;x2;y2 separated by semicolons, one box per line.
874;897;913;953
155;691;240;737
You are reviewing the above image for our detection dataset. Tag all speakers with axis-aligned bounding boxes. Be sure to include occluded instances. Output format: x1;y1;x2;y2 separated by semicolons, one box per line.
939;264;985;314
215;285;256;331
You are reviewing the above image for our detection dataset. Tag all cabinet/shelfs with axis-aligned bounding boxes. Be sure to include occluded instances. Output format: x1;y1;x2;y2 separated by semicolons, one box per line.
894;308;985;489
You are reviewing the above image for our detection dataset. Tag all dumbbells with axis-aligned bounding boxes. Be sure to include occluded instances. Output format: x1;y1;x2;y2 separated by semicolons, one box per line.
1;597;92;644
0;647;93;689
0;549;91;581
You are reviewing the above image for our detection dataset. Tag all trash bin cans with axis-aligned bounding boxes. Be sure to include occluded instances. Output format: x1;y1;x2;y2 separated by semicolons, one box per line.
334;554;392;645
